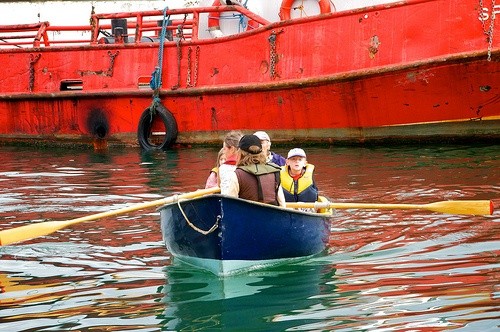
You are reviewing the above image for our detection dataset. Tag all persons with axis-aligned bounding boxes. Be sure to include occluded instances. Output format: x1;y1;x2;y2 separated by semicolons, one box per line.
204;130;319;212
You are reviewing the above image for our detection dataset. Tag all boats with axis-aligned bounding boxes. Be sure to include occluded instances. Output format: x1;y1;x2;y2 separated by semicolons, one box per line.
155;192;334;275
0;1;500;150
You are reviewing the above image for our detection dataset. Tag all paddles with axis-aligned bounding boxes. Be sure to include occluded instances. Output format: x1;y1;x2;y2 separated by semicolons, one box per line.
0;187;221;247
285;199;494;216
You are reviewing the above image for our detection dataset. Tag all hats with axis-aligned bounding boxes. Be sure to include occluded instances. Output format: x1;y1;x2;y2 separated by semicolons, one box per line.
239;135;262;154
253;131;270;142
287;148;306;159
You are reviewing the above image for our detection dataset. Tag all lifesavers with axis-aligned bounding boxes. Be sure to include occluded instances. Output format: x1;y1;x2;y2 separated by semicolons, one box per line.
137;103;178;153
207;0;259;40
279;0;331;22
